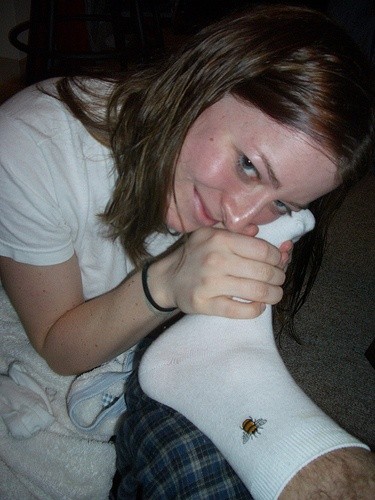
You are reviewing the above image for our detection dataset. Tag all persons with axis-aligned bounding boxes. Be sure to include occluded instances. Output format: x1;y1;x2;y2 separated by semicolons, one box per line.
140;208;375;500
0;10;374;499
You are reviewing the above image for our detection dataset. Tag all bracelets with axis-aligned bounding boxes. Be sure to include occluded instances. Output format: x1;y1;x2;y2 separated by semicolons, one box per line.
141;260;178;312
